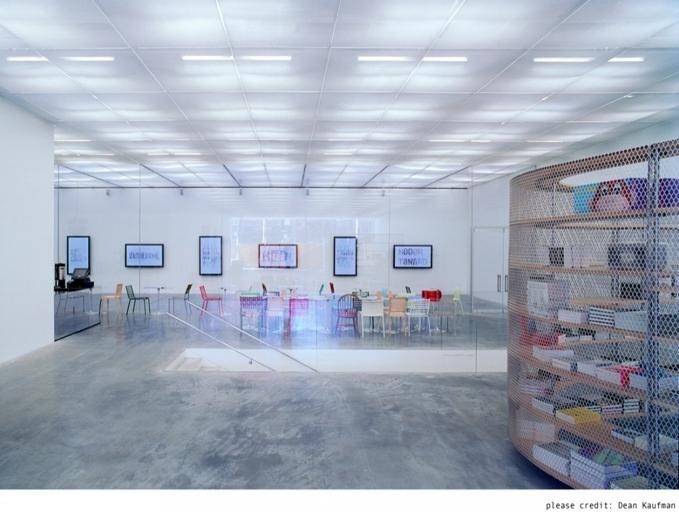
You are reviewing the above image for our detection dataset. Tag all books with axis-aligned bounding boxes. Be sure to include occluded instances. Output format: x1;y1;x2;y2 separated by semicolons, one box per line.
511;271;678;488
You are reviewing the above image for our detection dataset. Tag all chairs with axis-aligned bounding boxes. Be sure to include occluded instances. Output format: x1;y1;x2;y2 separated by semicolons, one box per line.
334;286;464;338
98;283;152;316
56;290;86;314
167;283;224;316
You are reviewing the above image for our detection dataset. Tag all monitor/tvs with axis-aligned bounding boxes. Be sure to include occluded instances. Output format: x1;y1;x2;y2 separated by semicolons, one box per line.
72;269;89;279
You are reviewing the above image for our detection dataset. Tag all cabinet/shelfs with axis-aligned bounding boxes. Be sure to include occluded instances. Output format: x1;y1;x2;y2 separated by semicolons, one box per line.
506;137;679;489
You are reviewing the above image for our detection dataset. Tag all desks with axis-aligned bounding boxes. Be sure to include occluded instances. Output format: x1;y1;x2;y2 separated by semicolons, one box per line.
54;283;94;313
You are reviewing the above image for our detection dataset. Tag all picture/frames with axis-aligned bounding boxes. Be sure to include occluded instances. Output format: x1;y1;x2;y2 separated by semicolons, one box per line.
257;243;299;269
125;242;165;268
334;236;358;277
198;235;222;276
393;243;433;269
66;235;91;274
